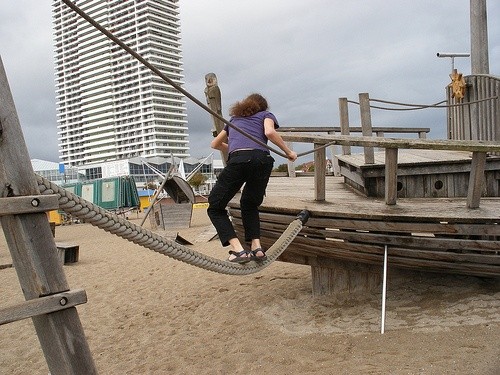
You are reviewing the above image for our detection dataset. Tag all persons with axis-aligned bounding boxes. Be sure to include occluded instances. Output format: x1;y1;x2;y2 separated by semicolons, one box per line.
206;92;298;264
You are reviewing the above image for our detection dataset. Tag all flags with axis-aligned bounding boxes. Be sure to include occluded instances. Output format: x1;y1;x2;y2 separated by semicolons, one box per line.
59;164;64;173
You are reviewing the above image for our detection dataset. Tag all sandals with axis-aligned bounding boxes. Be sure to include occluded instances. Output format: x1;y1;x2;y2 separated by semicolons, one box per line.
225;250;251;264
250;248;267;261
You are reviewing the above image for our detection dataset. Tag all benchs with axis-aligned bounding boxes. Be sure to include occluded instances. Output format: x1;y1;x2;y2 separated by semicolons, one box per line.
55;241;80;266
